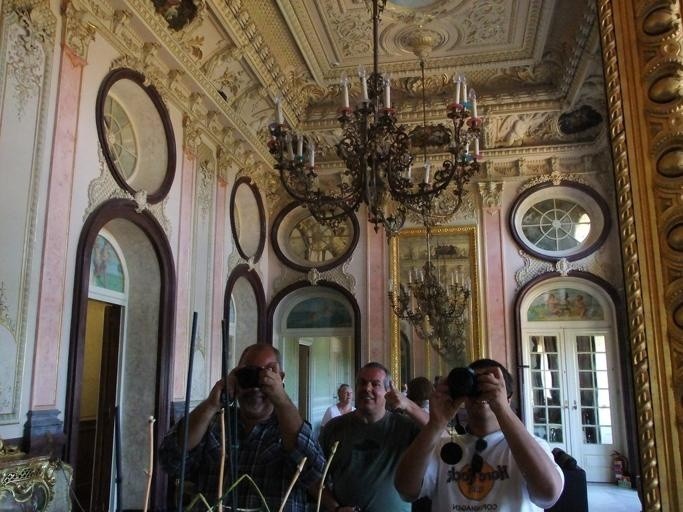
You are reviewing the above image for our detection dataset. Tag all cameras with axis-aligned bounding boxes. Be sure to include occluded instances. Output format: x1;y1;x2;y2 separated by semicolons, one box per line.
236;365;266;388
446;367;479;395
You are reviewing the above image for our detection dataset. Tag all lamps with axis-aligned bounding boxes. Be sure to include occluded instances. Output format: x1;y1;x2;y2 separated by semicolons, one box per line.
388;58;472;365
266;0;484;237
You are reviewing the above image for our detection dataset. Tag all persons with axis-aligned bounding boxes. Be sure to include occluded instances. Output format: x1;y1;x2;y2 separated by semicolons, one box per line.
403;376;432;414
318;383;358;428
393;358;567;512
150;343;326;511
316;361;444;511
